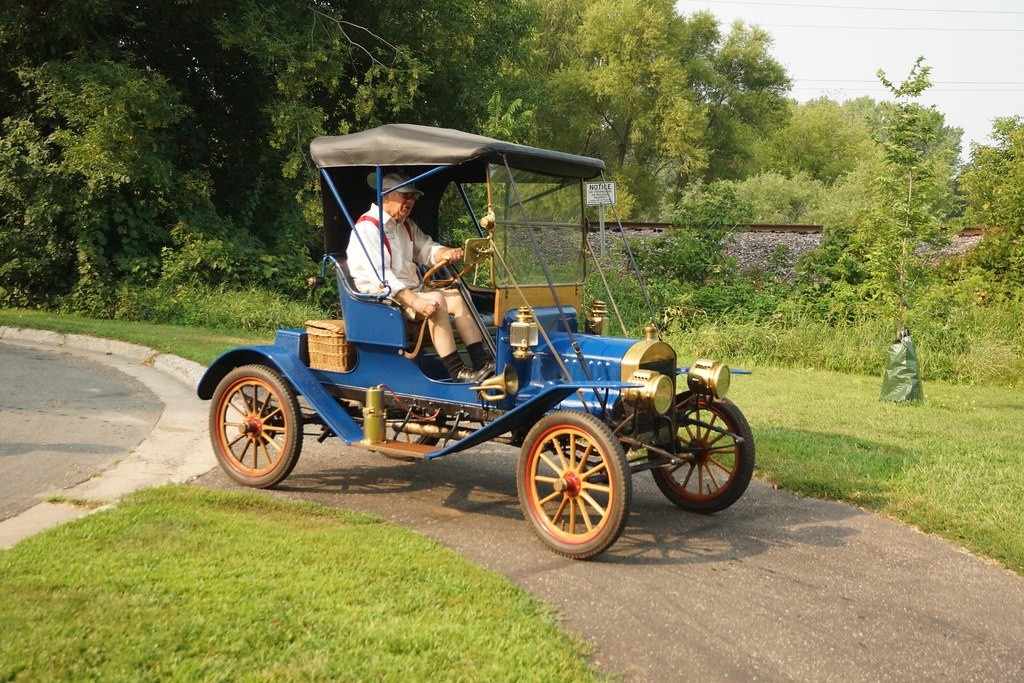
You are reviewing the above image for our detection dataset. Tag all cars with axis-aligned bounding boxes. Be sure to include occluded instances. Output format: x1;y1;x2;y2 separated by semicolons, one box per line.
195;122;755;562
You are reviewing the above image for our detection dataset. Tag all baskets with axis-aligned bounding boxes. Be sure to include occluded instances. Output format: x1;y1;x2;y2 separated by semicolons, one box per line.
305;318;357;371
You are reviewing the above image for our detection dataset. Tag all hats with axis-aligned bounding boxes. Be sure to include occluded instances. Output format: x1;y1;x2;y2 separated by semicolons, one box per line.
367;169;423;197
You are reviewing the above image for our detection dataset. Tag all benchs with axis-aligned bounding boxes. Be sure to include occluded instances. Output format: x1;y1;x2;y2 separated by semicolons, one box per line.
326;249;496;350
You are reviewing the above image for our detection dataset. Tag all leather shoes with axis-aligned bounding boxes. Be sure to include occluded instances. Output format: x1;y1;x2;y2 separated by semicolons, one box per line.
451;367;495;384
476;362;498;375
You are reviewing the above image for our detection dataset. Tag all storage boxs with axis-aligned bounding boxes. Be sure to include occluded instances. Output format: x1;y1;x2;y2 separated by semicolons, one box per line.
305;319;355;373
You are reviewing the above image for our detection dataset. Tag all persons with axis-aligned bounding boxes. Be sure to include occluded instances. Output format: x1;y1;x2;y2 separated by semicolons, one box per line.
347;173;497;384
